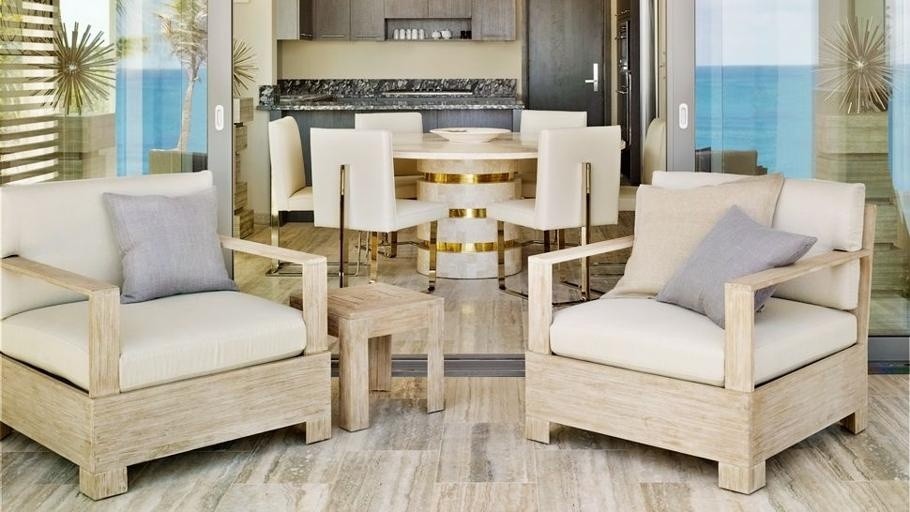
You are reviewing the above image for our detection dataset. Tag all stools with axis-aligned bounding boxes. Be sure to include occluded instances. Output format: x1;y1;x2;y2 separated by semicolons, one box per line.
288;280;446;433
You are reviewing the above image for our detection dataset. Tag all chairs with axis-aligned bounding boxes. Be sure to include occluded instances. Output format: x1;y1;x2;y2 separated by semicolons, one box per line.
352;112;427;259
307;125;451;295
2;171;334;502
483;123;622;304
517;110;592;250
524;170;878;494
269;113;371;279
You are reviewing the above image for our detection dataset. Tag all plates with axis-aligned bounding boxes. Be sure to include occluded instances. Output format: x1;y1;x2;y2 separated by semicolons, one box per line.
429;127;512;143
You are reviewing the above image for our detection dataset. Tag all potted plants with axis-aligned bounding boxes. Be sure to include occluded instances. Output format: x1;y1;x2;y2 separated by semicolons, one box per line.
146;0;209;173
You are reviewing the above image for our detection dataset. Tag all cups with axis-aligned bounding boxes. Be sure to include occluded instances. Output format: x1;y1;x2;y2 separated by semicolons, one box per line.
461;31;471;39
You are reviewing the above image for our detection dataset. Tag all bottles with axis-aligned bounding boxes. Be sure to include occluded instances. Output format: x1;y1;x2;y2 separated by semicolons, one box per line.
394;28;424;41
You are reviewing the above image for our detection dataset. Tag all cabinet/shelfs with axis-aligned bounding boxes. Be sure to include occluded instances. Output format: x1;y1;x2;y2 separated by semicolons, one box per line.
272;1;516;40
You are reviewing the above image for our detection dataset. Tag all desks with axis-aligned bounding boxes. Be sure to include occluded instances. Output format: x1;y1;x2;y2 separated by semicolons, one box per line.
392;126;626;281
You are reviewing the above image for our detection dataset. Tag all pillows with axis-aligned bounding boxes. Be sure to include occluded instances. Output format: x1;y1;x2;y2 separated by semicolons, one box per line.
599;171;818;331
102;185;243;304
